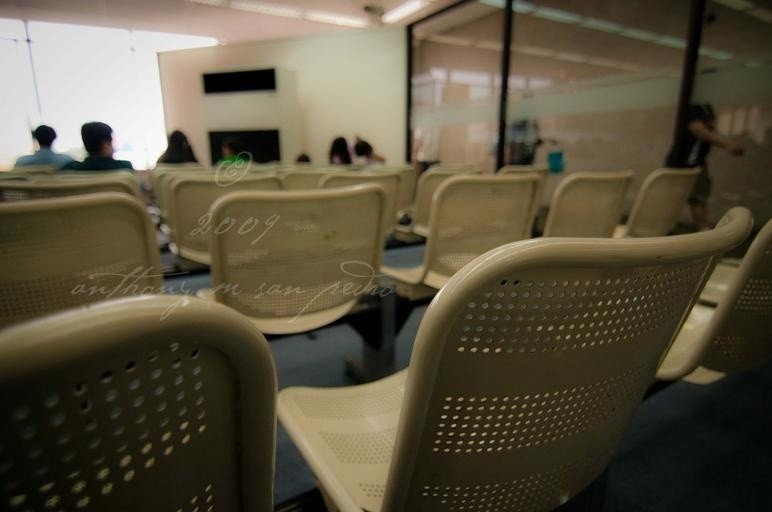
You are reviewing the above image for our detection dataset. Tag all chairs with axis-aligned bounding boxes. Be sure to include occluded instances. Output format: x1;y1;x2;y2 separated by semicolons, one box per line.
0;291;281;510
1;160;772;368
636;220;770;407
276;204;756;510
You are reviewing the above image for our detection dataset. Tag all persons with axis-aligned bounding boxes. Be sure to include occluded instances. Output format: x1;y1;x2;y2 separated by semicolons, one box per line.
412;126;443;176
216;138;252;163
156;131;198;162
503;117;542;238
16;125;74;168
665;66;747;234
330;138;384;166
73;122;132;170
